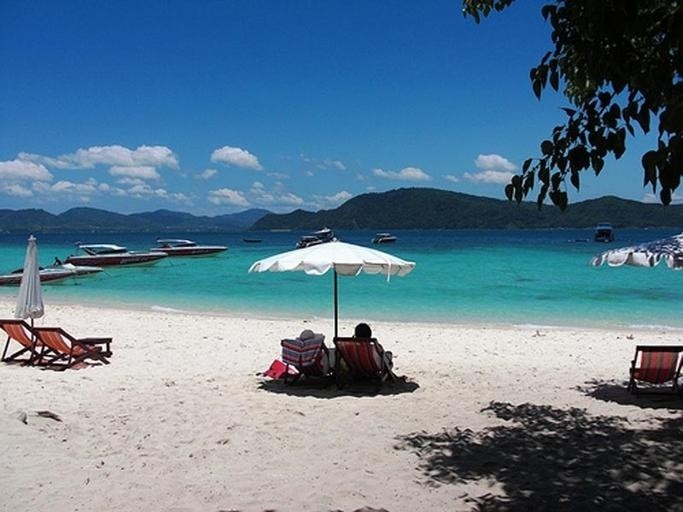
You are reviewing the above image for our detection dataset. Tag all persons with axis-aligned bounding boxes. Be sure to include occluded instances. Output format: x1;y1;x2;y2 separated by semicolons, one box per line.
298;329;328;376
355;321;392;366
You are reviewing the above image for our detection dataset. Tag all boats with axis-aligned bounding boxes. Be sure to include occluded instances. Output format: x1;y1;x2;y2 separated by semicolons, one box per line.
370;231;400;245
39;261;105;277
243;237;263;244
147;237;229;257
0;266;78;285
61;242;170;269
593;222;616;244
294;225;338;249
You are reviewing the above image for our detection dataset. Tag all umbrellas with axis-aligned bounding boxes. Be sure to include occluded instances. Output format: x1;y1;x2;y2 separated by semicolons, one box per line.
13;234;44;364
589;231;683;271
248;236;415;388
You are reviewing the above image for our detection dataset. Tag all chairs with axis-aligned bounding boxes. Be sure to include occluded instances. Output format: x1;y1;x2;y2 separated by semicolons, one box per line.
283;338;399;387
625;346;682;393
1;320;113;369
